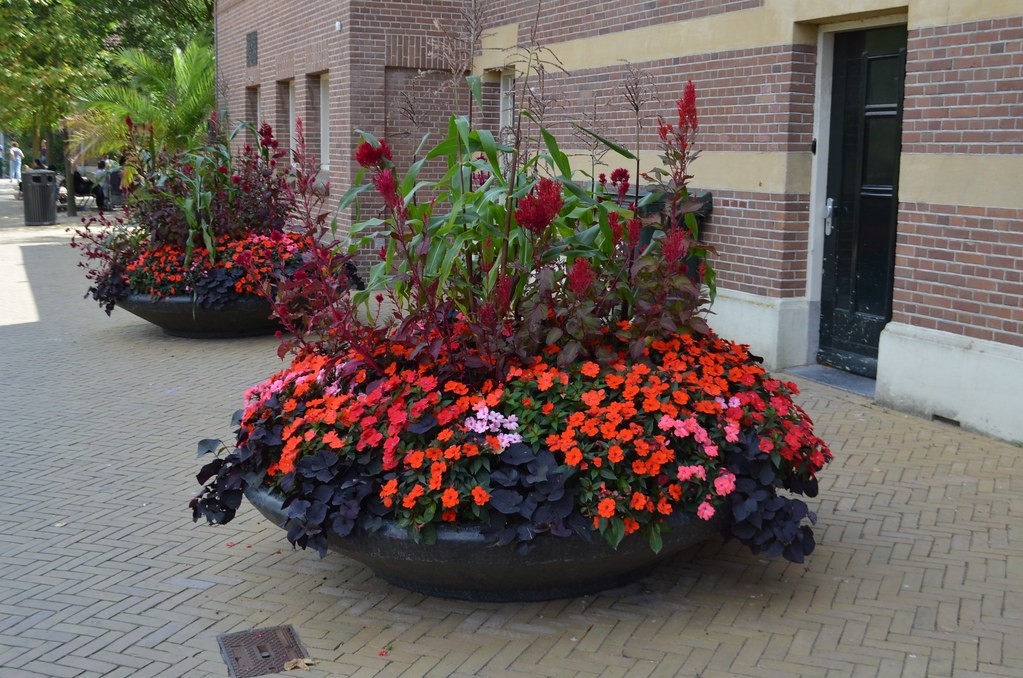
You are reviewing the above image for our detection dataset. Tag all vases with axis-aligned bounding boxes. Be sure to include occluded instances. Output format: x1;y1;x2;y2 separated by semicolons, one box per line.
240;480;729;604
110;293;316;339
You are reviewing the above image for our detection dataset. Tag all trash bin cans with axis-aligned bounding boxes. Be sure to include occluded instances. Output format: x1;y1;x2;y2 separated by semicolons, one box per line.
21;169;56;226
595;189;714;317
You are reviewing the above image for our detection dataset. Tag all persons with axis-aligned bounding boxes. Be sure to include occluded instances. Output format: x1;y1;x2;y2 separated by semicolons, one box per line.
68;161;115;210
13;158;45;200
8;142;24;183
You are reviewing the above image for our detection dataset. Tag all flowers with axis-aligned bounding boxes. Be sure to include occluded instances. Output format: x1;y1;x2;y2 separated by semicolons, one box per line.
65;0;831;564
